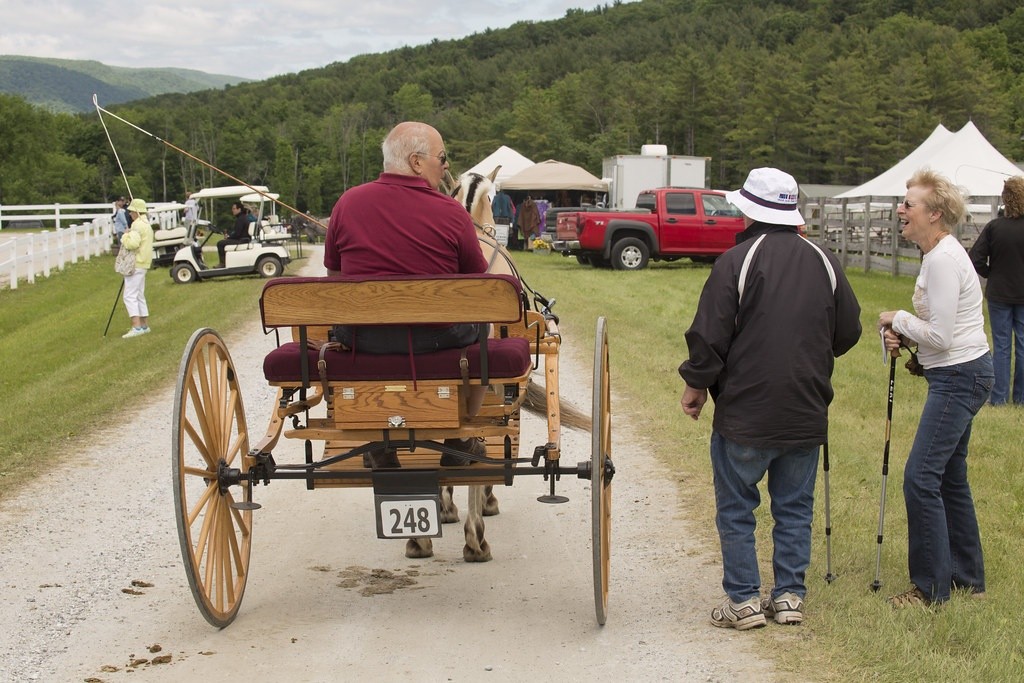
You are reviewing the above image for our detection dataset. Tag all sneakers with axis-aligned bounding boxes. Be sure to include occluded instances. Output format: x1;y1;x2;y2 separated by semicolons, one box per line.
760;590;804;625
886;585;932;612
142;326;151;334
121;326;145;339
709;595;767;631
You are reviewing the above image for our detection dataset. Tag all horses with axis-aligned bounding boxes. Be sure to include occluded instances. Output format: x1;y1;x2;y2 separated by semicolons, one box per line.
403;165;522;563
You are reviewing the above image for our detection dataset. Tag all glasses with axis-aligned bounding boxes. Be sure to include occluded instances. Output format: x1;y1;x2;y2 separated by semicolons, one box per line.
409;151;446;165
902;200;929;209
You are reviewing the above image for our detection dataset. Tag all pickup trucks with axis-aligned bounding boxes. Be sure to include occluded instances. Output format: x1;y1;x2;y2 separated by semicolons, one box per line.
551;187;809;271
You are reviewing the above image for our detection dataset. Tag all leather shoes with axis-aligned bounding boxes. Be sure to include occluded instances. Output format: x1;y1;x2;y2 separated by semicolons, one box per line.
440;436;487;466
362;441;401;468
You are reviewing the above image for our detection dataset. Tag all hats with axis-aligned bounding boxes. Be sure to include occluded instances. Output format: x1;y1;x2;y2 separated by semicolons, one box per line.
725;166;805;226
126;198;148;213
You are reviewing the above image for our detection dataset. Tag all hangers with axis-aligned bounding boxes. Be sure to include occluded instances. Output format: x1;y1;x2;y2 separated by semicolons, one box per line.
493;189;537;210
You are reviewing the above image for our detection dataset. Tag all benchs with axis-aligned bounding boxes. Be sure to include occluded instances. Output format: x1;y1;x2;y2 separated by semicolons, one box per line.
152;226;187;247
257;273;531;390
222;213;292;251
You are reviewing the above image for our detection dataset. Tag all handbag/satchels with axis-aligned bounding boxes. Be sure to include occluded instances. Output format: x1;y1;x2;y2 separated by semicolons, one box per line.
114;243;136;277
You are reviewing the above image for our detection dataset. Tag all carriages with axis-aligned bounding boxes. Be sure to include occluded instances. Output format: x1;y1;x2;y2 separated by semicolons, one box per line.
163;168;618;632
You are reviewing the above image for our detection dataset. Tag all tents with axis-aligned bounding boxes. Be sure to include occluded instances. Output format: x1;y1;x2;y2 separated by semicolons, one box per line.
831;121;1024;253
495;159;608;251
460;145;536;192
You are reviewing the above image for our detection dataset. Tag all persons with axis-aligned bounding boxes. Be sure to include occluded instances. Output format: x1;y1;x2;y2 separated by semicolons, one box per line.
120;198;153;338
244;203;257;222
677;167;862;631
877;169;995;610
324;121;490;467
213;202;251;268
968;176;1024;407
111;196;134;256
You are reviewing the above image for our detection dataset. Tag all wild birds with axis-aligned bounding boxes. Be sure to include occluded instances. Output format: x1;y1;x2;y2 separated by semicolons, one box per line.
291;210;331;243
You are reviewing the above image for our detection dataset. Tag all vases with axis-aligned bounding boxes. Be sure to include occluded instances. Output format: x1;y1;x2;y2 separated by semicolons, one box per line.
533;247;551;256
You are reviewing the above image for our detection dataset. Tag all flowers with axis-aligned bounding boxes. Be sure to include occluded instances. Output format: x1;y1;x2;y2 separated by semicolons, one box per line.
533;237;552;249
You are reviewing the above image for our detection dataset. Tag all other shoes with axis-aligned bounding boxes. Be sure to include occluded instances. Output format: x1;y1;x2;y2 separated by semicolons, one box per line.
213;262;226;268
